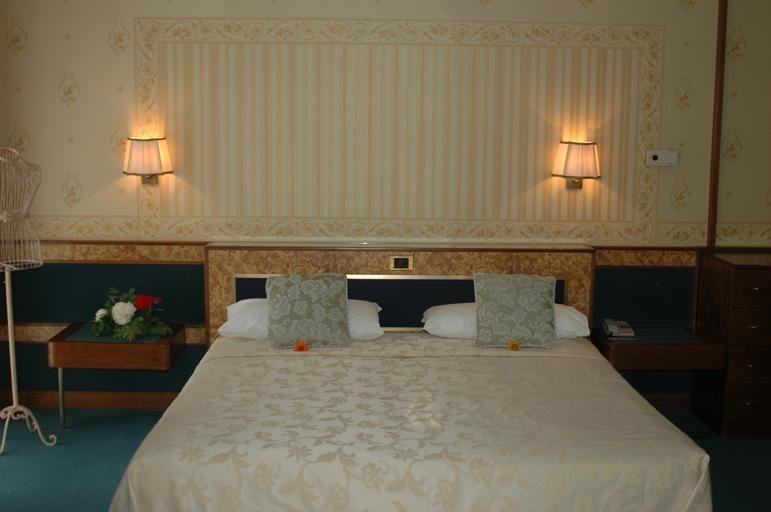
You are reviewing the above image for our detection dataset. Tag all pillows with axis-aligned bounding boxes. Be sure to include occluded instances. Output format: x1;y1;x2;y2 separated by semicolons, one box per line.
420;269;594;352
216;269;387;351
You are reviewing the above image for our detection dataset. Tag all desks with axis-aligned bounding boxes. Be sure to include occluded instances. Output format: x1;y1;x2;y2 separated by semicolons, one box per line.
47;322;188;428
600;324;722;373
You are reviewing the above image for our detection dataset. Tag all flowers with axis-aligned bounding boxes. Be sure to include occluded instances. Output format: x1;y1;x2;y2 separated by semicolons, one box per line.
93;284;174;342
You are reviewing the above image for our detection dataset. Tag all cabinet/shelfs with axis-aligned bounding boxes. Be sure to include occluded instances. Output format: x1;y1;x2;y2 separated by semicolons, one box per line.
692;254;771;432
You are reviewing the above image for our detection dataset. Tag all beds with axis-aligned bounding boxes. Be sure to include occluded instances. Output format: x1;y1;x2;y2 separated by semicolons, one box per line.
131;244;720;510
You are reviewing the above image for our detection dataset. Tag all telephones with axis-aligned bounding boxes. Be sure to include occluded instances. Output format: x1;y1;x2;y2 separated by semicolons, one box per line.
599;318;636;337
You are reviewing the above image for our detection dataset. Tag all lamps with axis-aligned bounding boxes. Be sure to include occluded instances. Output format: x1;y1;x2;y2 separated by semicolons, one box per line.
120;134;175;184
551;138;604;192
